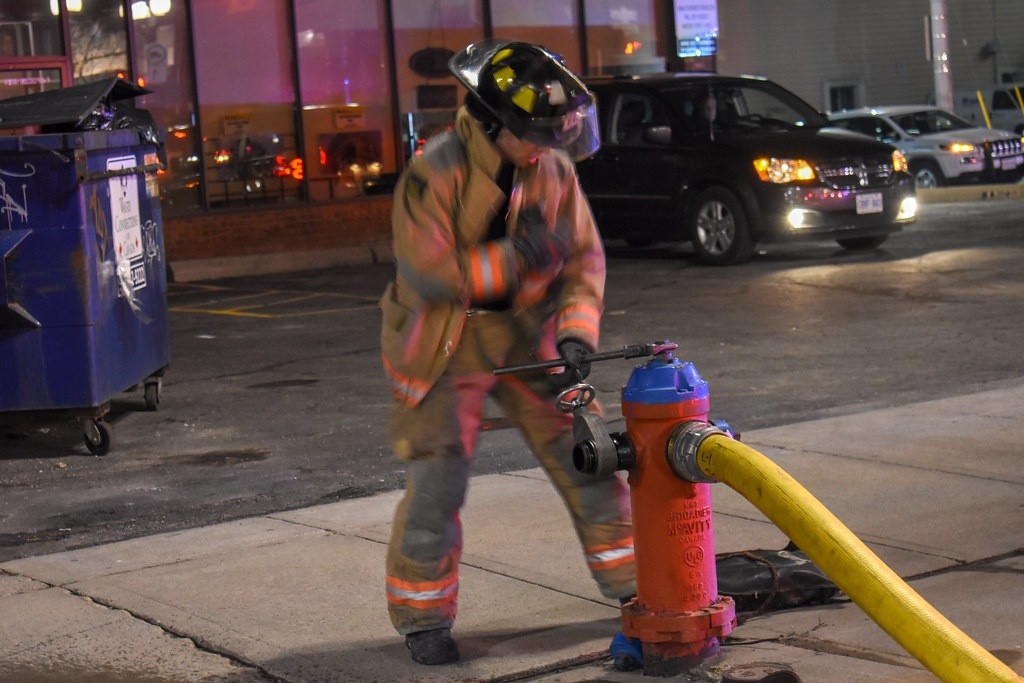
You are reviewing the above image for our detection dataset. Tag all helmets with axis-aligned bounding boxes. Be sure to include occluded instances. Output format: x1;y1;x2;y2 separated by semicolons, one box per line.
448;38;595;150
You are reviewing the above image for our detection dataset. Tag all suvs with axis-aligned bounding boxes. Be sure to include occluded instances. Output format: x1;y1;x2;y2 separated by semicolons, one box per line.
575;71;919;267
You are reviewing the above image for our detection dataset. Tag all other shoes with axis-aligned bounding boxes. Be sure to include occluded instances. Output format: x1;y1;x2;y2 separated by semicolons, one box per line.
405;627;459;664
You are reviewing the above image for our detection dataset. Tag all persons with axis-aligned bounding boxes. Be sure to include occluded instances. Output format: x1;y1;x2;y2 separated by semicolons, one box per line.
379;42;637;664
694;91;722;141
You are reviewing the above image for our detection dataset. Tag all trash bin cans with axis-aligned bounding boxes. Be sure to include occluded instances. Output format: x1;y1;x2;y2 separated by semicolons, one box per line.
0;76;171;456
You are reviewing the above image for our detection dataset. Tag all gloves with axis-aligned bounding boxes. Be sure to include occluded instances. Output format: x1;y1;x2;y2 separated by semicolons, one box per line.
547;342;590;389
516;204;572;271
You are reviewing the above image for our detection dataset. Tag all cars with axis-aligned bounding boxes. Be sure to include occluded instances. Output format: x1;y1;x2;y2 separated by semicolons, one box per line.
829;104;1024;189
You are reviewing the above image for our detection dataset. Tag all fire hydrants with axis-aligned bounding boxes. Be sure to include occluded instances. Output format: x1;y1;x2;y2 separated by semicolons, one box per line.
571;340;741;678
